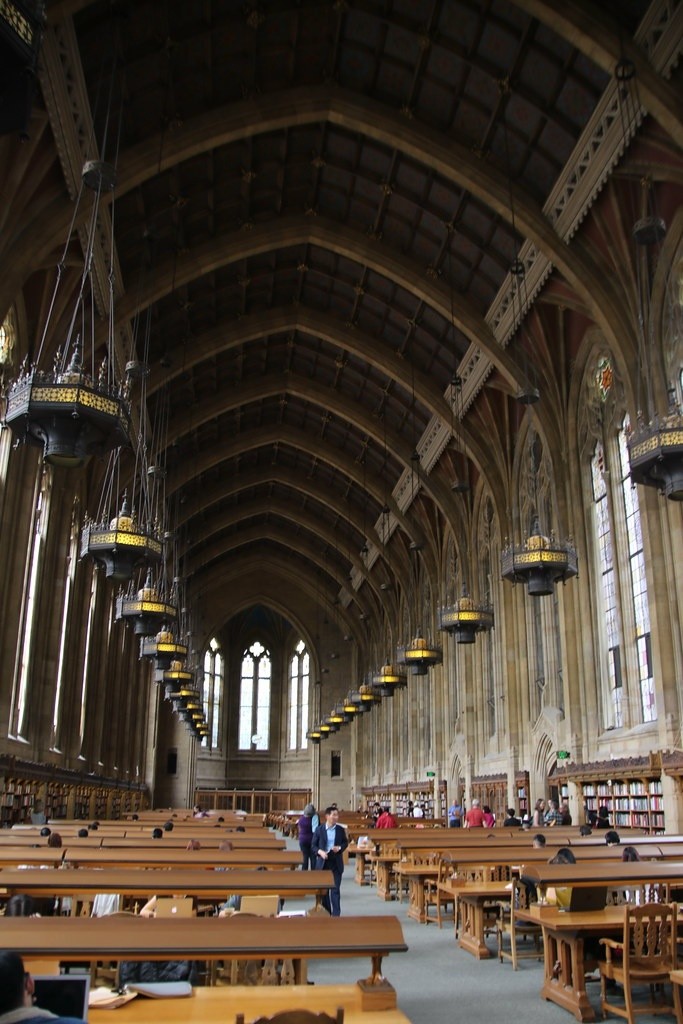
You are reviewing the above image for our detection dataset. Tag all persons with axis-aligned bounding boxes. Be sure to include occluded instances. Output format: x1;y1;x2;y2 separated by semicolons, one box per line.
132;815;138;821
448;799;572;827
152;805;245;851
357;801;425;845
0;952;88;1024
139;895;198;917
311;807;348;916
622;847;658;904
532;806;620;847
218;866;281;917
31;799;46;825
298;805;320;870
0;895;40;917
18;822;100;869
545;849;576;906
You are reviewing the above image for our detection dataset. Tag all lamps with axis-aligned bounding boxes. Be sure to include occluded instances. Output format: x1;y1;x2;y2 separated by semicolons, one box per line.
5;0;683;746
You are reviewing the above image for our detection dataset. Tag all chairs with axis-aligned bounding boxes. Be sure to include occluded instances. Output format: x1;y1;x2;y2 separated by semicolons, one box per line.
597;901;683;1024
59;894;125;988
495;877;545;971
457;865;491;882
211;913;307;986
606;882;671;906
423;859;456;929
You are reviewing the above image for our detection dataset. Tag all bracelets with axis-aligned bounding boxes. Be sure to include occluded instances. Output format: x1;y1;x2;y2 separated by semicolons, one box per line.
339;846;341;850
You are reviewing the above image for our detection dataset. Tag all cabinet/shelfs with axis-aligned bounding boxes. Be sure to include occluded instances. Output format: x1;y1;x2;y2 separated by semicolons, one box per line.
0;753;151;830
360;749;683;837
194;787;312;814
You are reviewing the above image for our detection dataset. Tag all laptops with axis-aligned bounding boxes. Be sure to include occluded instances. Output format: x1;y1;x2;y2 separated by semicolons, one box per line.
559;885;608;912
32;973;91;1021
240;895;279;918
156;898;193;918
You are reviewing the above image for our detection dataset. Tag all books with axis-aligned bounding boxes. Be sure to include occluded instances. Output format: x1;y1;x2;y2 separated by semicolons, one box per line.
0;783;141;829
562;782;665;835
88;982;191;1009
367;793;446;819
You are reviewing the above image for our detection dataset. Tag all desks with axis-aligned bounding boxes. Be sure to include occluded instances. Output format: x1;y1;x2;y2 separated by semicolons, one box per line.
0;808;683;1024
